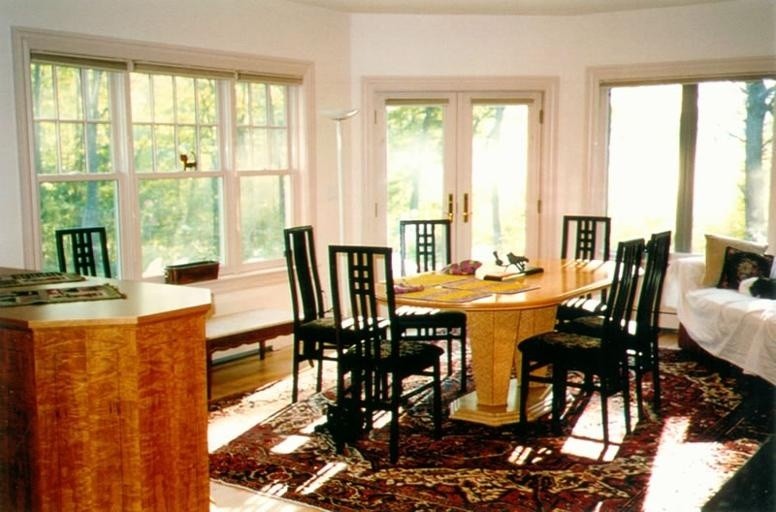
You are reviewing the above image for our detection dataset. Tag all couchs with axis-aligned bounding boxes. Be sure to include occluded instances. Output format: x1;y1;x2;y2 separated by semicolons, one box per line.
678;254;776;390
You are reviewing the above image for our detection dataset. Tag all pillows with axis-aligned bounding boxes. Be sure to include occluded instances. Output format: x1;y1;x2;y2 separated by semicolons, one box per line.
717;245;776;296
704;234;771;289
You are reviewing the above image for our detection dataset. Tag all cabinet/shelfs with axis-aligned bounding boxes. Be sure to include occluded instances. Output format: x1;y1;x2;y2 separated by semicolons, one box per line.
0;267;215;512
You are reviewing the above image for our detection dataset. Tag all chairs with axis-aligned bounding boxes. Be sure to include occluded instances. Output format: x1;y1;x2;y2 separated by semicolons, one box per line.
55;227;112;280
394;219;468;392
515;238;645;445
555;230;672;422
556;215;611;324
327;244;444;468
284;226;384;403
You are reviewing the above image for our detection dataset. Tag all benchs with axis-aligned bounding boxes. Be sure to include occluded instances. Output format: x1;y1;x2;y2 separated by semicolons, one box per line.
205;307;315;397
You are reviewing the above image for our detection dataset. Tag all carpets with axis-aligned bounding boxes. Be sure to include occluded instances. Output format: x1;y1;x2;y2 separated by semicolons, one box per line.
209;318;776;512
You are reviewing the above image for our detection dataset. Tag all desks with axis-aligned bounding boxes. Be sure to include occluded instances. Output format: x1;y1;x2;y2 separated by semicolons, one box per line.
371;255;647;428
